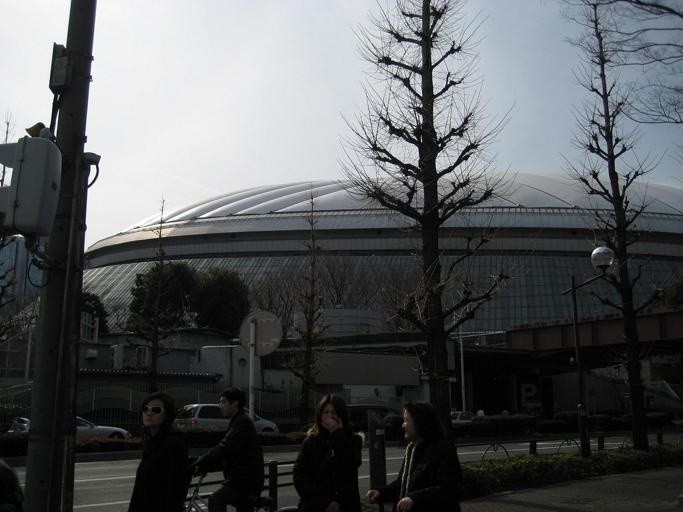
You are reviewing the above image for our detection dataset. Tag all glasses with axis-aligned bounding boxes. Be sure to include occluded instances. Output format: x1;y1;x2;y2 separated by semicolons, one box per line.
142;406;165;414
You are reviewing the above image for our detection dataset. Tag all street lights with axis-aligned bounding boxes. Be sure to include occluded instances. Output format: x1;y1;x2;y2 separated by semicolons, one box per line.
558;245;613;456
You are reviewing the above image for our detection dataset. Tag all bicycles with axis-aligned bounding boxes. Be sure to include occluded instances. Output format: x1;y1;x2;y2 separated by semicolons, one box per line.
186;469;298;511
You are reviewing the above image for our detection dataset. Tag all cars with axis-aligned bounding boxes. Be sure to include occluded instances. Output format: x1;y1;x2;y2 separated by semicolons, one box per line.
74;416;130;442
450;410;475;425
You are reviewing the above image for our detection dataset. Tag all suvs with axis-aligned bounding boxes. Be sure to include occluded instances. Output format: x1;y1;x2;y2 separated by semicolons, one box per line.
174;403;279;441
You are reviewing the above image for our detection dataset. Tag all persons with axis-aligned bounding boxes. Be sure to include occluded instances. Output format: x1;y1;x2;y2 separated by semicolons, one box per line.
126;389;192;510
0;458;24;511
291;391;362;509
364;400;463;511
190;385;264;510
